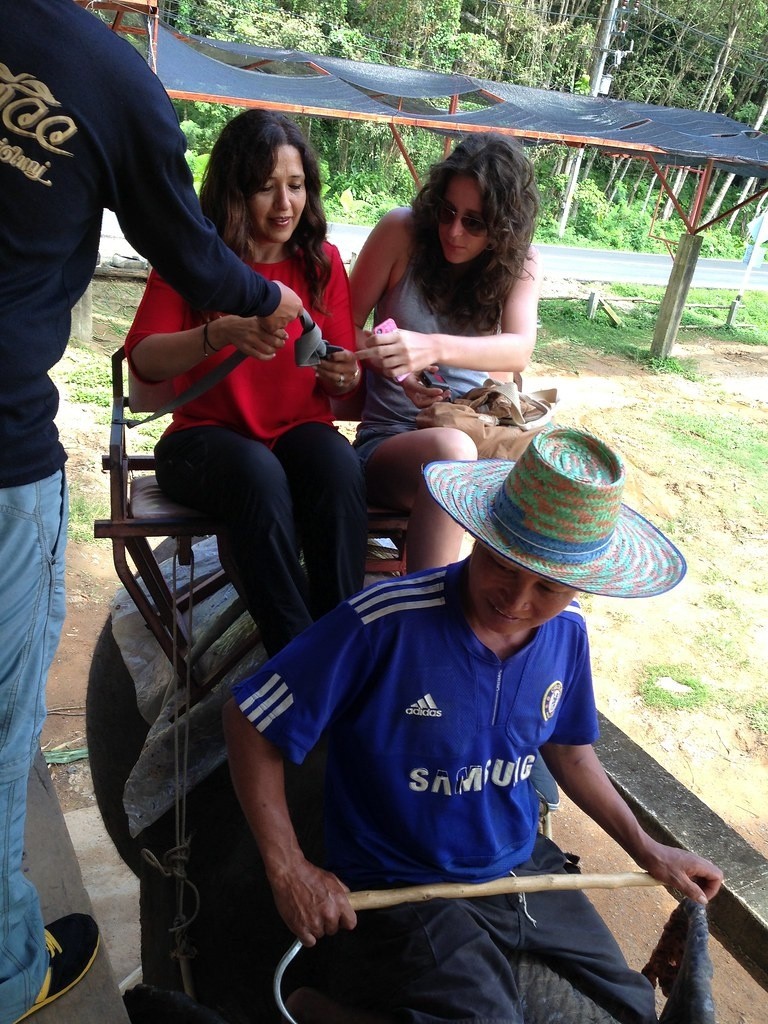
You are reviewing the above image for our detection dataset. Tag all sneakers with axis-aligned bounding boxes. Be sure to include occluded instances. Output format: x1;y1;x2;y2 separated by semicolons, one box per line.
8;912;100;1024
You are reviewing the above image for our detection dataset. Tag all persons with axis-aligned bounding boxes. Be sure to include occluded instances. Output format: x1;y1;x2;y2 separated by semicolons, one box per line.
124;107;363;658
230;432;723;1024
1;0;302;1024
350;133;543;578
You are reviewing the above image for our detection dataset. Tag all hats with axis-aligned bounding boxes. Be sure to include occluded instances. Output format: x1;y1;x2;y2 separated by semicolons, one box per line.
423;426;687;597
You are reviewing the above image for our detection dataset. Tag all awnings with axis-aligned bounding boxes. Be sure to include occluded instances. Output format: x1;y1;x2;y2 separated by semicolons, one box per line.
154;31;768;173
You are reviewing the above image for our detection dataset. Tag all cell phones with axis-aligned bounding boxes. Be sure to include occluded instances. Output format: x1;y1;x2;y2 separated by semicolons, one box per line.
373;318;412;383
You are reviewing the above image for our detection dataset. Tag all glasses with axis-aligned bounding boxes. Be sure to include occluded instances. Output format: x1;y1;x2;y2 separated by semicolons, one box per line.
434;197;489;237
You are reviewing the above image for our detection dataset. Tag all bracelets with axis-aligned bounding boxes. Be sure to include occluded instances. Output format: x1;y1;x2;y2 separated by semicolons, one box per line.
203;322;219;357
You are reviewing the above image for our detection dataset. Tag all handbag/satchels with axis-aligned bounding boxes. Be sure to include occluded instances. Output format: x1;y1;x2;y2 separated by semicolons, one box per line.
416;374;562;462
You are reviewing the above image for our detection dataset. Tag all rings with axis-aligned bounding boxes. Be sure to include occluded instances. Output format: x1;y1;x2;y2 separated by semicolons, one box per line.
336;374;344;387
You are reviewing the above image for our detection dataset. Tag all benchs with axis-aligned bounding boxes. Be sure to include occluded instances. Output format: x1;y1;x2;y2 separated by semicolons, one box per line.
94;344;522;725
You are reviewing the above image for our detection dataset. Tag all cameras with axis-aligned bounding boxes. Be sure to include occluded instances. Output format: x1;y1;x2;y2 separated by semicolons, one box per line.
421;370;449;391
